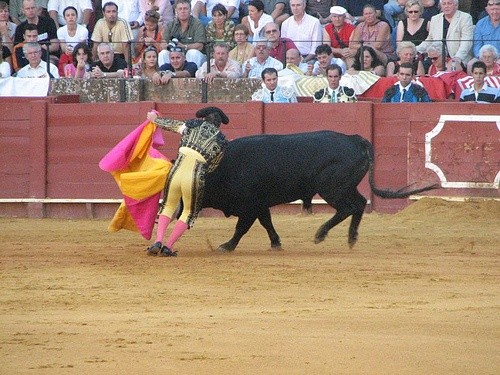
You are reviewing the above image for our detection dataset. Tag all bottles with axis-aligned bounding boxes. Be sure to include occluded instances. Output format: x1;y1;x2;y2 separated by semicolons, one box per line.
331;90;337;103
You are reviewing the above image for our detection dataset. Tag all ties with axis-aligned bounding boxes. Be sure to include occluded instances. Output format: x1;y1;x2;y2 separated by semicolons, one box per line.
332;91;335;102
270;92;275;101
402;89;406;100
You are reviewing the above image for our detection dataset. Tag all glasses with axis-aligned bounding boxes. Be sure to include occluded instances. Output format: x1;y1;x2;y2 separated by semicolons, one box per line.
108;32;112;42
265;30;277;34
170;47;182;52
146;12;159;20
431;57;439;61
489;2;500;6
408;10;420;13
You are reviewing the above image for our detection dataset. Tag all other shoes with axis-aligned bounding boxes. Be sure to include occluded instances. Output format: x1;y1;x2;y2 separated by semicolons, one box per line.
147;242;162;256
159;246;178;257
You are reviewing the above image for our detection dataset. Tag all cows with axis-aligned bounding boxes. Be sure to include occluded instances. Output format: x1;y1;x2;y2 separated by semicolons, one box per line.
170;130;441;254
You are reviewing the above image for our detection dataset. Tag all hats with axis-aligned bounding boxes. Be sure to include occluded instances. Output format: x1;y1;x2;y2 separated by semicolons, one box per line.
330;6;347;15
196;107;229;125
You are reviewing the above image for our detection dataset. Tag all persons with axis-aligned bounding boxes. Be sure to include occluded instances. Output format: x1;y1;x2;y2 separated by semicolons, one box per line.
0;0;500;104
144;107;232;257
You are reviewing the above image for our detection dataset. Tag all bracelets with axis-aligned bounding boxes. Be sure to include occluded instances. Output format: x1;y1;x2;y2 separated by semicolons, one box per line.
102;72;106;78
